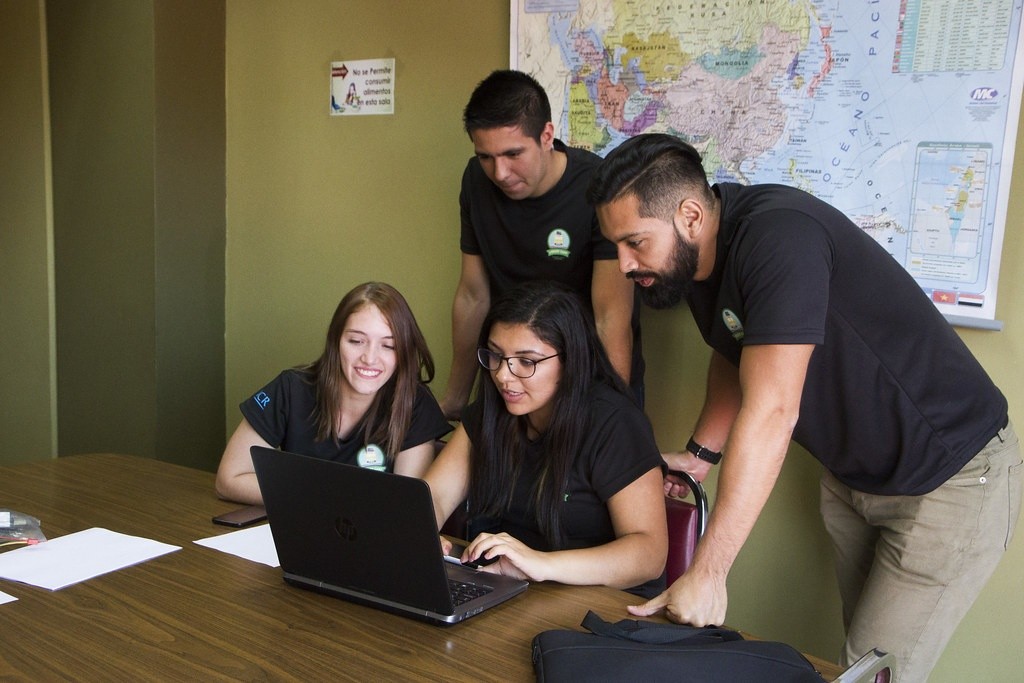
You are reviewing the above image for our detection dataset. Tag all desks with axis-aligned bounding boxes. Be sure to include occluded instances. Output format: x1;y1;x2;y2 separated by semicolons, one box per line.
0;453;852;683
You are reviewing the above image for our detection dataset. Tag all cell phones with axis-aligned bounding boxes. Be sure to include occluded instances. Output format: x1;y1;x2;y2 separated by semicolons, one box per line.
213;504;268;527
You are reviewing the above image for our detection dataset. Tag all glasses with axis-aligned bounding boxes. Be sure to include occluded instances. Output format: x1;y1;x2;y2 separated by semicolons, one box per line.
477;348;558;378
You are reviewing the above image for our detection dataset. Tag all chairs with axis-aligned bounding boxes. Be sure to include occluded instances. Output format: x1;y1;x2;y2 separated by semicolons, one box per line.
665;469;708;588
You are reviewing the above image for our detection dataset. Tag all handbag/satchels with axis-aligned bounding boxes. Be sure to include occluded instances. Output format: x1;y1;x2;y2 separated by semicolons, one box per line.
531;610;826;683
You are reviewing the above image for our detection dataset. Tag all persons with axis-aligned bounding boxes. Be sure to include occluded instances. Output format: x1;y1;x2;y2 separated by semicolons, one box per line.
215;282;455;508
421;281;669;600
436;69;645;422
587;132;1023;683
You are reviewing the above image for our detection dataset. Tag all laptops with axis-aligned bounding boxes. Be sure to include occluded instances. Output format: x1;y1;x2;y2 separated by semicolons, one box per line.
250;446;530;623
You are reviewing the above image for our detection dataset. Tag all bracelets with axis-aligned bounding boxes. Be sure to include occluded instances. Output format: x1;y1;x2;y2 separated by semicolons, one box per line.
684;438;724;466
